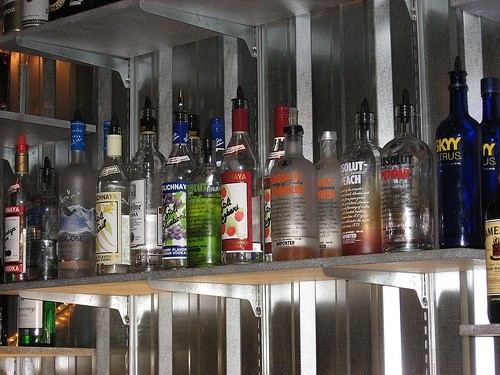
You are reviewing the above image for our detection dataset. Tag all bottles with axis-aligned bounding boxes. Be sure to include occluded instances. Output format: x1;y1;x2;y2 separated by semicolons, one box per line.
263;88;436;262
17;295;55;347
1;85;263;284
437;56;500;248
0;0;84;34
484;178;500;324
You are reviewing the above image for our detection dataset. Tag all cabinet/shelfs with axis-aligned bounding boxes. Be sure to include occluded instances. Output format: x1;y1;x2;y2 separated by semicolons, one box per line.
0;0;500;375
